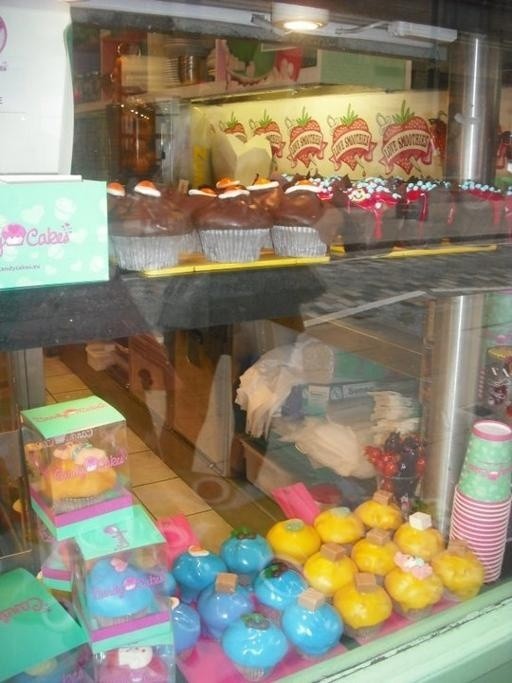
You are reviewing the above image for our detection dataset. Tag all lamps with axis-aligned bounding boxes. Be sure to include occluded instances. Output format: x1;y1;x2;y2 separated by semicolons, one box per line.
269;1;331;34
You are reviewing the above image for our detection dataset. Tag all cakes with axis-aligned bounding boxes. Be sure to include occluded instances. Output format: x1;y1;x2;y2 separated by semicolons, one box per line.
100;649;168;683
429;183;458;242
86;559;153;622
343;178;405;246
105;183;127;211
216;177;243;194
109;181;192;238
249;176;286;213
177;187;218;222
275;180;342;250
197;185;269;231
504;188;512;235
455;181;503;241
43;443;115;503
397;185;429;245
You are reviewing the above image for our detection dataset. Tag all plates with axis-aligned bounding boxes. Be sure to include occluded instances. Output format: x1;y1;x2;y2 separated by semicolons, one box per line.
330;245;497;259
149;56;180;93
142;257;331;277
160;37;203;50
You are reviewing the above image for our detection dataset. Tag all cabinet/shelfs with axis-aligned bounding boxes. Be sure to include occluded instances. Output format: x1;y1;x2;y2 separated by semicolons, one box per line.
127;324;247;478
0;172;512;683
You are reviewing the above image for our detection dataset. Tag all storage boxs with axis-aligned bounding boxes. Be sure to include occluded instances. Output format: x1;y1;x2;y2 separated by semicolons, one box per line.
0;179;109;291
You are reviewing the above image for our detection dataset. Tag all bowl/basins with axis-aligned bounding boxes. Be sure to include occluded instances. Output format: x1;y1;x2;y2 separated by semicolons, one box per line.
179;55;207;85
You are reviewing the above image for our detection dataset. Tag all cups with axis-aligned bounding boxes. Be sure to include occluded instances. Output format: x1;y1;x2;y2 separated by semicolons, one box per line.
111;234;181;272
198;230;269;264
271;226;328;256
377;475;422;499
182;231;196;254
448;421;511;584
